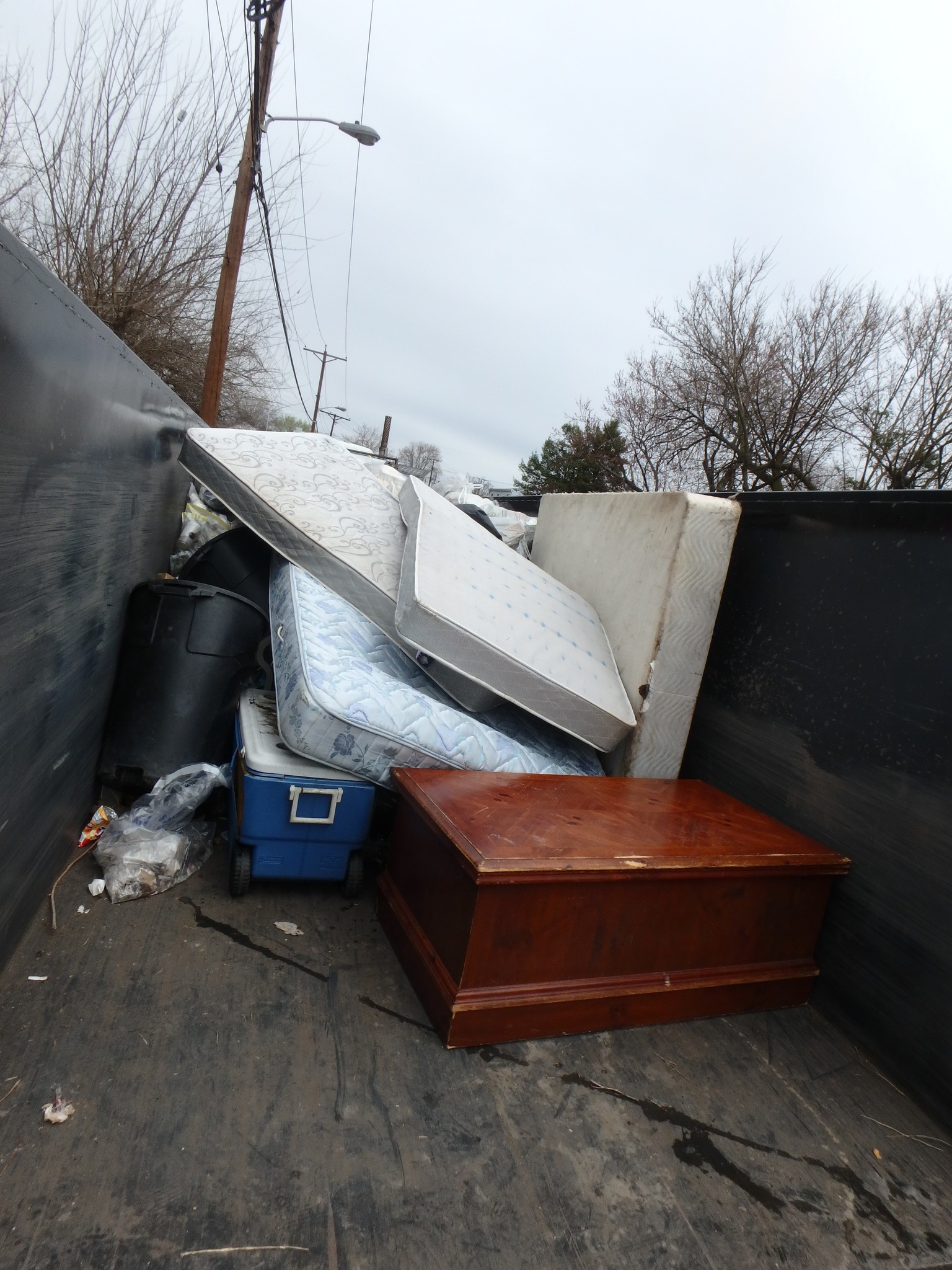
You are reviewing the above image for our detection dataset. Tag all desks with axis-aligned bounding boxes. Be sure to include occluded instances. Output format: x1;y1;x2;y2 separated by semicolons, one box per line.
372;767;852;1048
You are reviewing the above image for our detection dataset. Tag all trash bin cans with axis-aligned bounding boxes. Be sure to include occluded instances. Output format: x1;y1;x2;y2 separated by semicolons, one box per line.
96;577;268;805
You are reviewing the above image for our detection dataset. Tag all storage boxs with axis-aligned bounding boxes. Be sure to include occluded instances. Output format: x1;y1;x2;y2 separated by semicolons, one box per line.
231;689;376;898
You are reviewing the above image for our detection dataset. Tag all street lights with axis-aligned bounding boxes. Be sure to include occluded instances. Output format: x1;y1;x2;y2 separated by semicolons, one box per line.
199;115;382;428
310;406;347;433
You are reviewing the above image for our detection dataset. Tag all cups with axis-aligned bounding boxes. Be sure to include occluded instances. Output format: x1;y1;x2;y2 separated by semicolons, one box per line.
176;522;202;550
